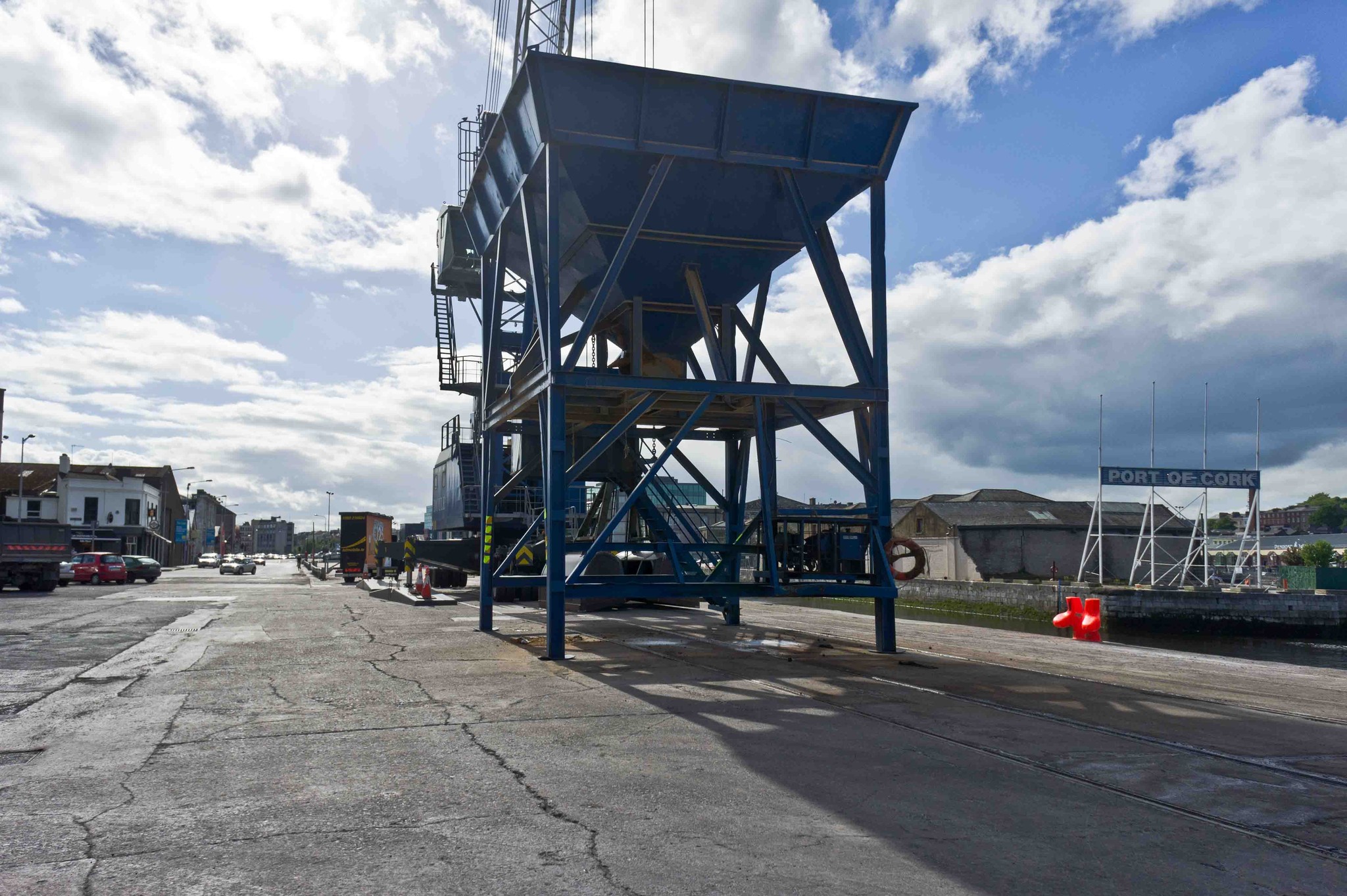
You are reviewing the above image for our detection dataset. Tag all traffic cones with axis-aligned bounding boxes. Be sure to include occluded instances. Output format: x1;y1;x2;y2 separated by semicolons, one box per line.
410;563;424;594
416;569;437;599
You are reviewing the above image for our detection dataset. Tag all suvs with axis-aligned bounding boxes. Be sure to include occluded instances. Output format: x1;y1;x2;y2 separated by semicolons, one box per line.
220;557;256;575
197;553;219;568
122;555;162;584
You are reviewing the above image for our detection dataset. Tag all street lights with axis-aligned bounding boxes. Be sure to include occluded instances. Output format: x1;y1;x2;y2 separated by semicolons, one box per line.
314;514;326;556
186;480;212;565
326;491;334;534
207;495;227;552
17;434;35;522
161;467;194;566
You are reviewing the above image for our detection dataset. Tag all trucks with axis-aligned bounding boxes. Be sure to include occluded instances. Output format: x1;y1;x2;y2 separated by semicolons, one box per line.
0;516;72;591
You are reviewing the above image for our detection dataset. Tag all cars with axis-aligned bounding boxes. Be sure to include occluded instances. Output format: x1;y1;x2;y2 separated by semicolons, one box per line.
296;552;340;558
224;552;295;566
70;552;127;585
57;561;75;586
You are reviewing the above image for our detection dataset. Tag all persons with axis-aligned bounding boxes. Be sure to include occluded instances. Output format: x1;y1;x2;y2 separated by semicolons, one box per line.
296;554;302;569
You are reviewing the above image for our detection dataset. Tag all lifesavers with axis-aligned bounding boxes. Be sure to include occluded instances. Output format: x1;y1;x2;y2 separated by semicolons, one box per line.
885;537;926;581
